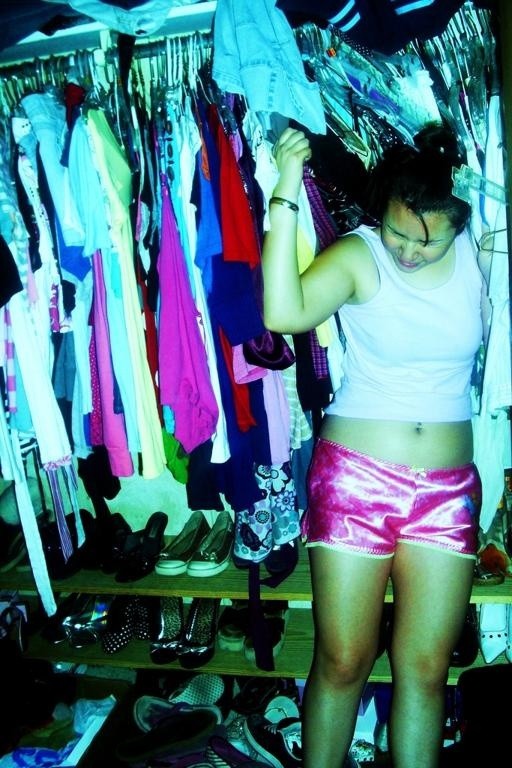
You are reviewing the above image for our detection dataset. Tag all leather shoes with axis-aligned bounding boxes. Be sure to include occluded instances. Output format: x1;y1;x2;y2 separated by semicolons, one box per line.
10;595;291;667
449;602;512;666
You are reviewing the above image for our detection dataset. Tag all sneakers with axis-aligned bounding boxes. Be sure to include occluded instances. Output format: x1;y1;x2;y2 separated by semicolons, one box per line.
244;714;303;768
132;673;225;734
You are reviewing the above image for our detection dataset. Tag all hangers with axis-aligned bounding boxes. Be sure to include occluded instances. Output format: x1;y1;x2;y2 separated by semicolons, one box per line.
292;2;500;124
0;0;249;126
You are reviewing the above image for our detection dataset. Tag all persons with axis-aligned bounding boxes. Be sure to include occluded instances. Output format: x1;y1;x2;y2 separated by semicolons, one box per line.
258;124;492;767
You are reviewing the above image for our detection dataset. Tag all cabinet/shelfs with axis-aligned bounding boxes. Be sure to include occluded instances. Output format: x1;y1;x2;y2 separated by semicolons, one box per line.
1;536;512;688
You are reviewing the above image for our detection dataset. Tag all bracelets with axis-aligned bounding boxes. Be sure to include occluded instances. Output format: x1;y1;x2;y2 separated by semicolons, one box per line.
266;196;298;212
